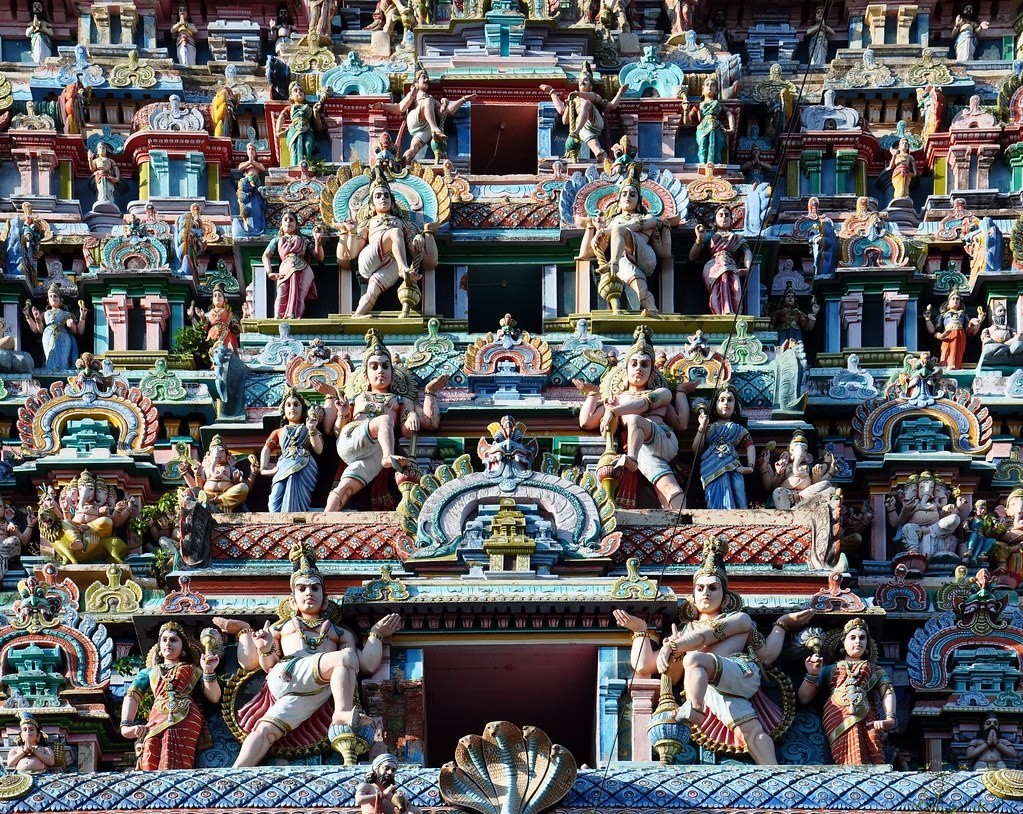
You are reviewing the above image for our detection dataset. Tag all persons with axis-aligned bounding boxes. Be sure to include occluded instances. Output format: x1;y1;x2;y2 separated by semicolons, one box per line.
0;0;1022;594
118;622;224;772
965;713;1017;770
7;712;57;774
799;615;898;765
212;543;403;768
352;754;423;814
611;532;818;765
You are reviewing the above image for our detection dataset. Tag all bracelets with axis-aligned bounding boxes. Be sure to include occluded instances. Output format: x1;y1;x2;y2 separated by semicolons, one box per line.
631;631;651;640
256;642;277;656
118;719;134;728
22;748;27;754
31;748;35;753
668;640;677;659
202;672;217;683
772;621;789;631
370;631;384;642
804;673;819;684
884;711;898;730
984;741;990;747
236;628;255;637
994;740;1000;746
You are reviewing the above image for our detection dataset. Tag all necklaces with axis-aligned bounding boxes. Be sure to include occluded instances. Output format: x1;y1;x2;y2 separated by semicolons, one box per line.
157;662;184;691
841;658;868;684
290;611;331;656
